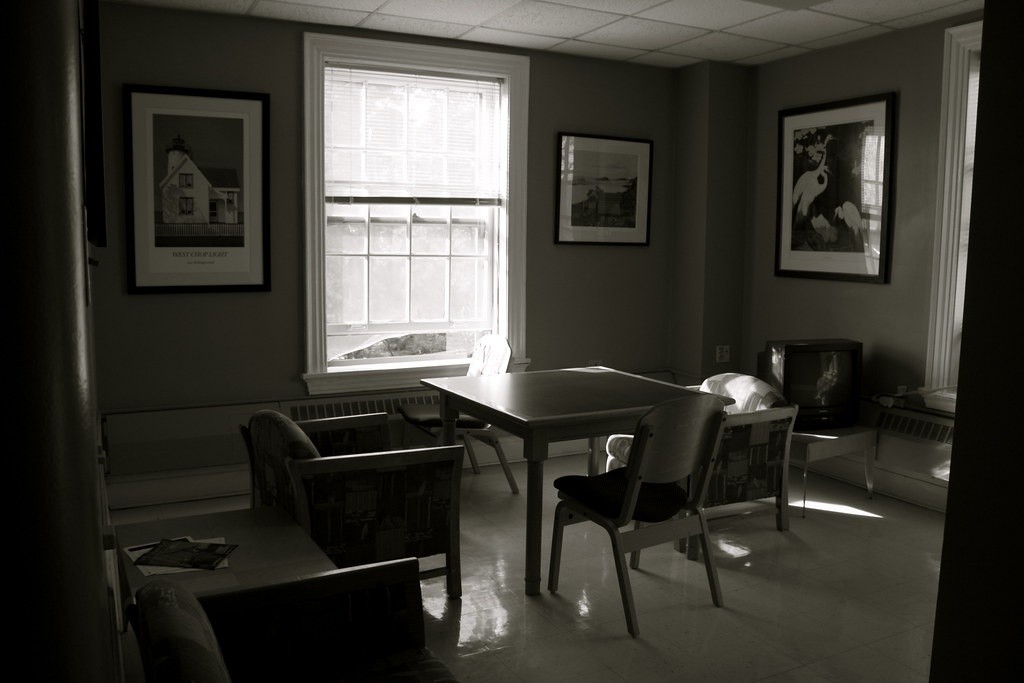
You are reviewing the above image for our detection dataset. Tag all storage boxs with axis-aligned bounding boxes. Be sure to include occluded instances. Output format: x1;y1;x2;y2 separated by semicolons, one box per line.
924;391;956;412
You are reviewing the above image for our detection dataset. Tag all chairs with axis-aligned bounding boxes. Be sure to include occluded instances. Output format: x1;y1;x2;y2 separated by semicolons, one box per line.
547;394;730;640
399;333;520;493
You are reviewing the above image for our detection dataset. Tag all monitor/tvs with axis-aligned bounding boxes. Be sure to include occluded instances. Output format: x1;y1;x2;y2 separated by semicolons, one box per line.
755;338;865;432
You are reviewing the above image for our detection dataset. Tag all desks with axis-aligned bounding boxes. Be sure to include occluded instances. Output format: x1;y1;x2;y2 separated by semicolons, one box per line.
788;426;878;517
419;366;735;596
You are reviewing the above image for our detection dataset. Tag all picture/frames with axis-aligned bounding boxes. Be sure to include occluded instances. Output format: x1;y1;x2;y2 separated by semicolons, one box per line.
553;131;654;245
124;83;271;293
774;90;899;285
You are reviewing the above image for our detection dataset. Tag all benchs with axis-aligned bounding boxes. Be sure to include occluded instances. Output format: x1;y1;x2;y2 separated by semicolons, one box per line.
135;556;460;683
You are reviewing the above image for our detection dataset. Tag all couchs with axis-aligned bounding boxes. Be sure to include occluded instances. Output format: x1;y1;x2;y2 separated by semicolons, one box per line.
239;410;462;601
606;373;798;551
